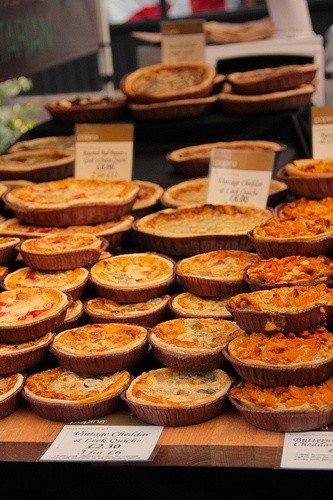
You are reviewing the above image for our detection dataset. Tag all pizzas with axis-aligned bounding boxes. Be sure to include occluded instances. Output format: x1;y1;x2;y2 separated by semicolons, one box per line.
0;63;333;430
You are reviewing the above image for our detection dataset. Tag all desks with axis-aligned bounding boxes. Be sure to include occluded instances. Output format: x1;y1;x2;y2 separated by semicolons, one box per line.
137;30;325;109
